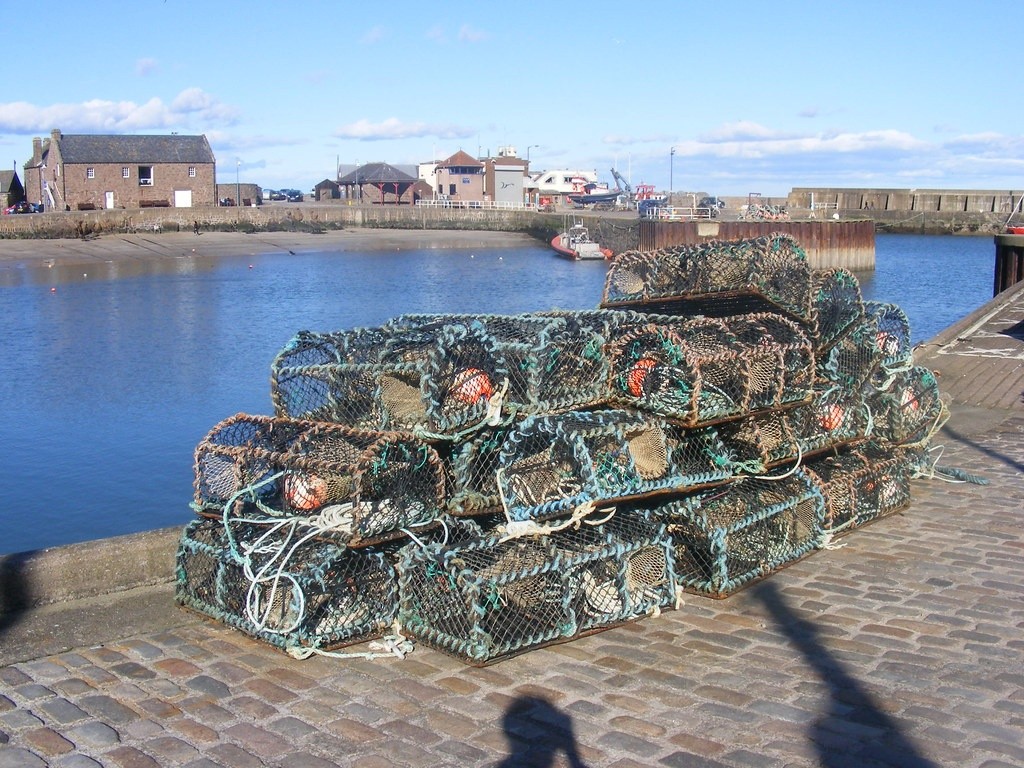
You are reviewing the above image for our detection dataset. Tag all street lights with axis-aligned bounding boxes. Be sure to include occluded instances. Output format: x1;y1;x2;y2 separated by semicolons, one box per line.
236;160;242;208
670;145;675;209
527;145;539;159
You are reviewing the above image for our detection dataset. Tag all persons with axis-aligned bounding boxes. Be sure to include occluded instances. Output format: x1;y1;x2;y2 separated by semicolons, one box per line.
153;224;162;234
193;221;202;236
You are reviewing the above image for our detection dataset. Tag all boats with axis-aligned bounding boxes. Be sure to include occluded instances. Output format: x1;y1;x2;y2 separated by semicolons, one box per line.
569;189;624;206
551;211;614;261
1008;226;1024;235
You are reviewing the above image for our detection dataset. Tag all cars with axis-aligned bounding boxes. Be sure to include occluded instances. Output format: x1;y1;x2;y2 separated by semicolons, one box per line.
639;199;671;220
695;196;725;217
262;187;304;203
